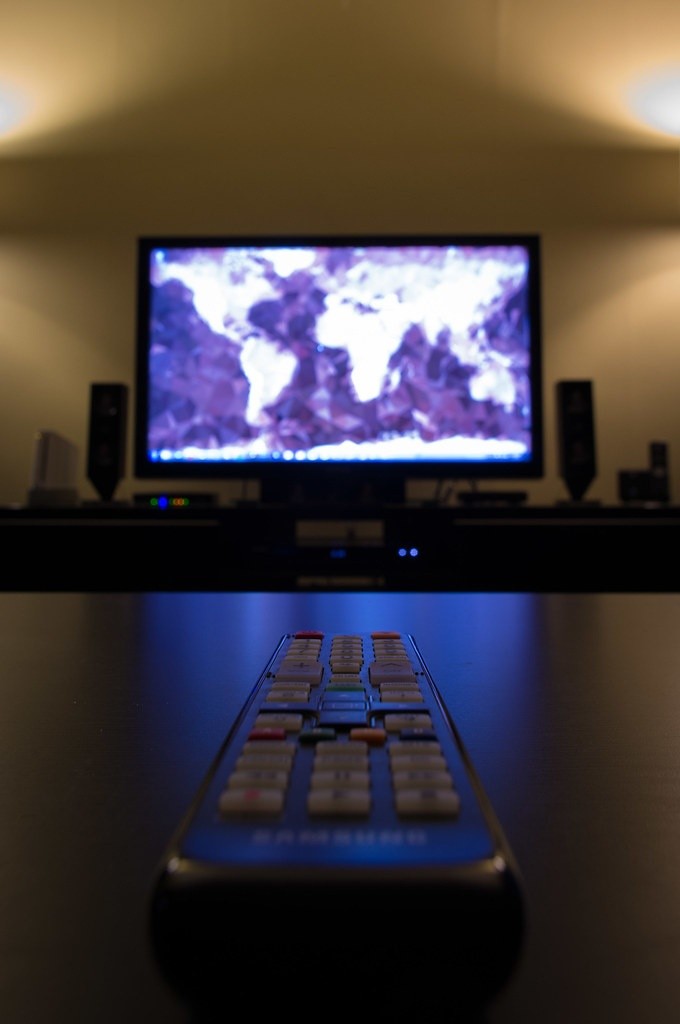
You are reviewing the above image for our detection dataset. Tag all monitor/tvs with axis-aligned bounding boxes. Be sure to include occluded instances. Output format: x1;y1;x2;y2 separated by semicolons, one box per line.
134;237;546;517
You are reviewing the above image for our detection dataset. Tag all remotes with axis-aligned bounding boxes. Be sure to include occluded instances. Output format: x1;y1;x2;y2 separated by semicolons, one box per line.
143;630;528;990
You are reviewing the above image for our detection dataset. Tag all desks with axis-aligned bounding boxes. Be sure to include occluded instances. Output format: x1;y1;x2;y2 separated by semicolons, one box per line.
0;590;680;1024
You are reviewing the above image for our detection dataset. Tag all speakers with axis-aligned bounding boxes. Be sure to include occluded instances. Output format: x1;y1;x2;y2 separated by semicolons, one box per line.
551;379;600;508
84;384;127;508
